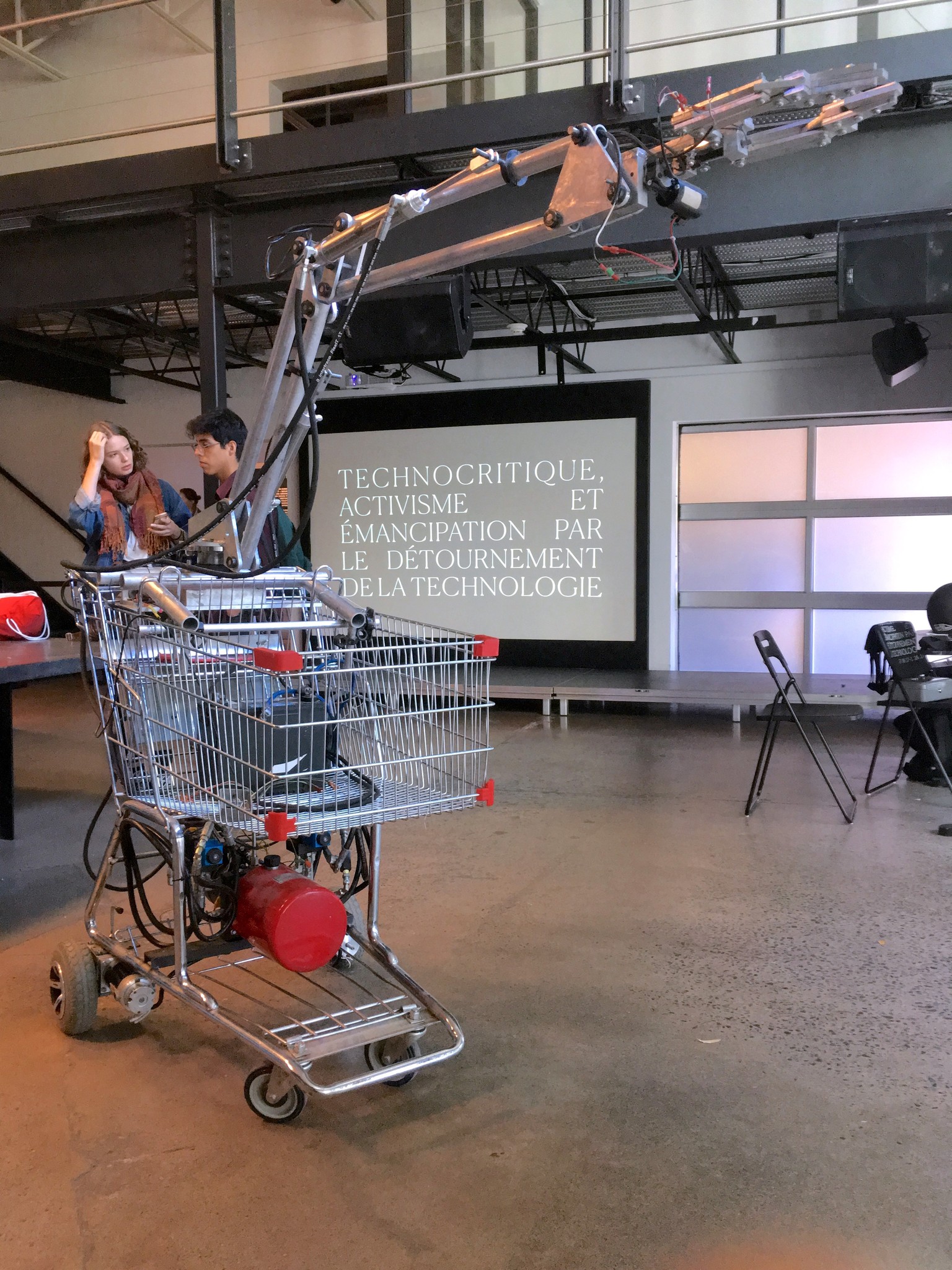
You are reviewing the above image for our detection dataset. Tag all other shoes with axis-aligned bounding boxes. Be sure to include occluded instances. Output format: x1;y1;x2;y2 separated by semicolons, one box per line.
156;773;174;790
130;765;149;792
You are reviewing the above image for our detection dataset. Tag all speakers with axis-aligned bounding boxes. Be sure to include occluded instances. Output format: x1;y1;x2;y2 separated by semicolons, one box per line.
871;321;929;388
338;267;476;368
836;209;952;322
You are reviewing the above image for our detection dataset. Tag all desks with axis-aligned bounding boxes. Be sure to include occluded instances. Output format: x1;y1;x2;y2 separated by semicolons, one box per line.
1;635;284;841
916;629;952;667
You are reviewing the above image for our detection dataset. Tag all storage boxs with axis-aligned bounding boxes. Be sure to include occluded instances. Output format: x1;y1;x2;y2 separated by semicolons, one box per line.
888;677;952;702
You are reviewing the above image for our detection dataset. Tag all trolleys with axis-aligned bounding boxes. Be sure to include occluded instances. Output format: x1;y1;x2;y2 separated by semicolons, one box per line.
48;563;502;1127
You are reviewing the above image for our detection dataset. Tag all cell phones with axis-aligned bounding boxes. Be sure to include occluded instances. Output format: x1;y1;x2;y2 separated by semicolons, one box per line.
152;512;168;532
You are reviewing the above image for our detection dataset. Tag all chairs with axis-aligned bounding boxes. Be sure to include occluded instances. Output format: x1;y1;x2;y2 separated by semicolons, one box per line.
744;630;864;824
865;619;952;795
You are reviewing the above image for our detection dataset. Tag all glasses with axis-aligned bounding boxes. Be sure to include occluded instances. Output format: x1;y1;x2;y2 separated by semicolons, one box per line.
191;443;222;454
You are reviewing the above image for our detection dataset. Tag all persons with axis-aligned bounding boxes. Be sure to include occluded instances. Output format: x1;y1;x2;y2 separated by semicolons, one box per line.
69;408;311;661
